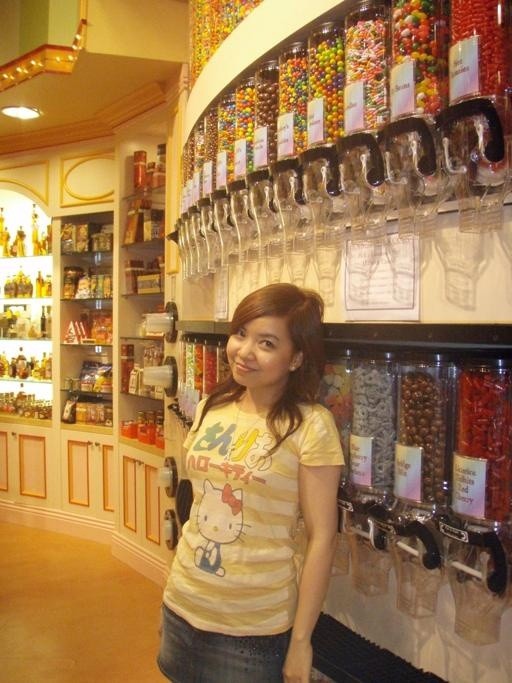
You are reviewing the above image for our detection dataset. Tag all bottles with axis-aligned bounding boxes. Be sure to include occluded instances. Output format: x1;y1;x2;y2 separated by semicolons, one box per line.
133;143;166;193
1;204;53;419
136;410;165;449
62;266;112;300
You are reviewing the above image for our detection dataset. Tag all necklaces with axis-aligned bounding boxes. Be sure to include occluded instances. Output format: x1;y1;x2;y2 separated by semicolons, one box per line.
223;392;269;460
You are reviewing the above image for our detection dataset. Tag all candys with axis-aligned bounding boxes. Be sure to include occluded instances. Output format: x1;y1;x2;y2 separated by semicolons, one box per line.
175;340;232;395
308;342;512;523
180;0;511;202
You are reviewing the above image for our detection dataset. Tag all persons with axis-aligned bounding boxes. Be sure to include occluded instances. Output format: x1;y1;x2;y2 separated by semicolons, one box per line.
153;282;349;682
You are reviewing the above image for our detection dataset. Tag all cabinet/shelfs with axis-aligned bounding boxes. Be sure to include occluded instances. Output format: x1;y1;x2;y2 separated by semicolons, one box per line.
0;0;164;590
